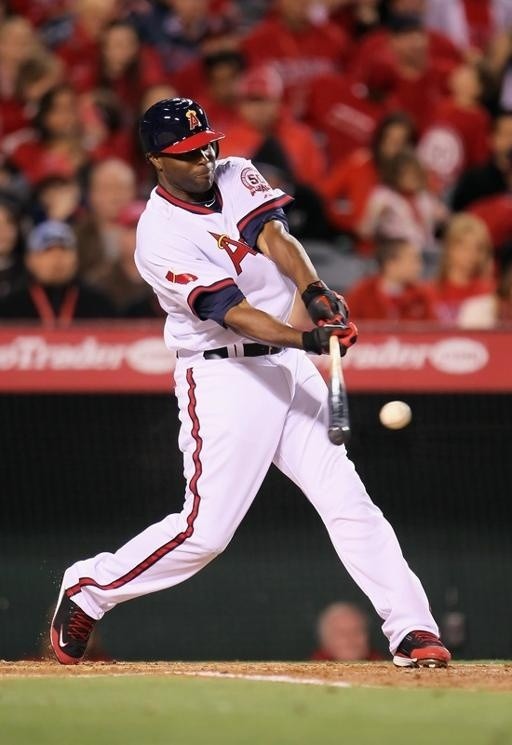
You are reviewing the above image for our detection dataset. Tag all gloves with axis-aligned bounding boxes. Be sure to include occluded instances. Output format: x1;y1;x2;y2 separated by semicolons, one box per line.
301;279;349;326
302;320;358;357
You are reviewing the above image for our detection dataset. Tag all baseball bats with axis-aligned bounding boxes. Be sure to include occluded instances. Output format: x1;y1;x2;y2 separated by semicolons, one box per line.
327;336;350;447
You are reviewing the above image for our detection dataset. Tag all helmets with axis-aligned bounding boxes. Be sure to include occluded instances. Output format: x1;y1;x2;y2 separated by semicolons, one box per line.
139;97;225;159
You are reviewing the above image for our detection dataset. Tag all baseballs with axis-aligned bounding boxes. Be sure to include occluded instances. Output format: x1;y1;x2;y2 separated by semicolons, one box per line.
381;401;410;427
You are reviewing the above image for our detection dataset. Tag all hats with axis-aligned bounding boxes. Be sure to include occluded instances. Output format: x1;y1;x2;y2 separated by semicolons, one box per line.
25;219;77;252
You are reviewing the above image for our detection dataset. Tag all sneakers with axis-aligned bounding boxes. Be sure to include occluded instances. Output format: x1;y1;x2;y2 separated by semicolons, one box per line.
392;631;451;669
50;567;97;665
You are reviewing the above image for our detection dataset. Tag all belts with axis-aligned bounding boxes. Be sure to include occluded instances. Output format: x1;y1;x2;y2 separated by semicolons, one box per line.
204;342;283;359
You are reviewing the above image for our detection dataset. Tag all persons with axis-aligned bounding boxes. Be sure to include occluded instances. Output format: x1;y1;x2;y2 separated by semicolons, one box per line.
51;94;453;672
1;0;510;331
30;606;113;662
310;600;383;661
440;611;480;660
